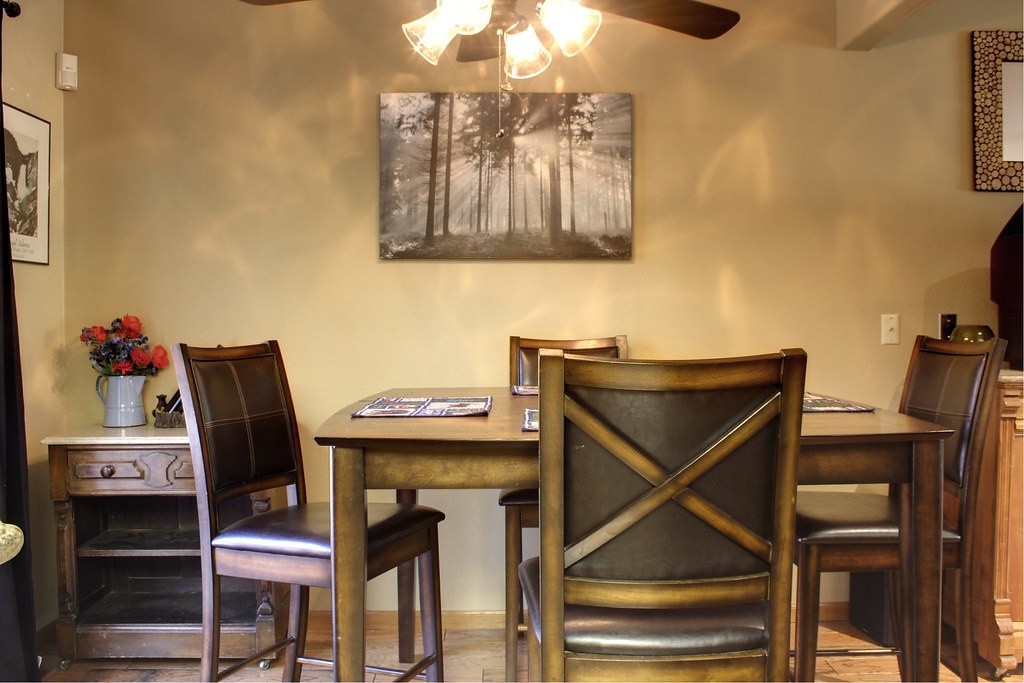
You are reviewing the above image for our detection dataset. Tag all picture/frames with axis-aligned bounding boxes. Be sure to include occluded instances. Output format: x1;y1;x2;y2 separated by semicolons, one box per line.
970;30;1024;192
3;102;51;266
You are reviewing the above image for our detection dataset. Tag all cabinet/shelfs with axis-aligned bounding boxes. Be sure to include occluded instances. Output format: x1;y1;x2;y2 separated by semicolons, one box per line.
41;428;290;671
949;369;1024;681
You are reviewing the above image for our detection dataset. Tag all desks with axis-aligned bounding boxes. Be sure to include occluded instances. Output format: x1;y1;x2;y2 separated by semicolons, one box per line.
315;387;959;683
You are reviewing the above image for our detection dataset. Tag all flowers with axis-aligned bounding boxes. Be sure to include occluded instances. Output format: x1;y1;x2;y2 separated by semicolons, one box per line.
79;314;170;376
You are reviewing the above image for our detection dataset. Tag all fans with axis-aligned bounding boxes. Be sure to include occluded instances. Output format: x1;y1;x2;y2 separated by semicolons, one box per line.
244;0;743;65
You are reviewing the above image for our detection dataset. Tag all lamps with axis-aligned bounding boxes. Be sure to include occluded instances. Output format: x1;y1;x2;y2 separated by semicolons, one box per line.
400;0;603;81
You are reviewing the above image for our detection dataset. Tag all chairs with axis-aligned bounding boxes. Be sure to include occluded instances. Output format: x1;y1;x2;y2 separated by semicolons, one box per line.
175;340;447;683
526;348;809;683
499;333;633;683
790;334;1009;683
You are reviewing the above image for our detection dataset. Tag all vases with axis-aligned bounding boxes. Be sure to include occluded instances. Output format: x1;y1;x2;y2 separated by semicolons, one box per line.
95;374;148;429
